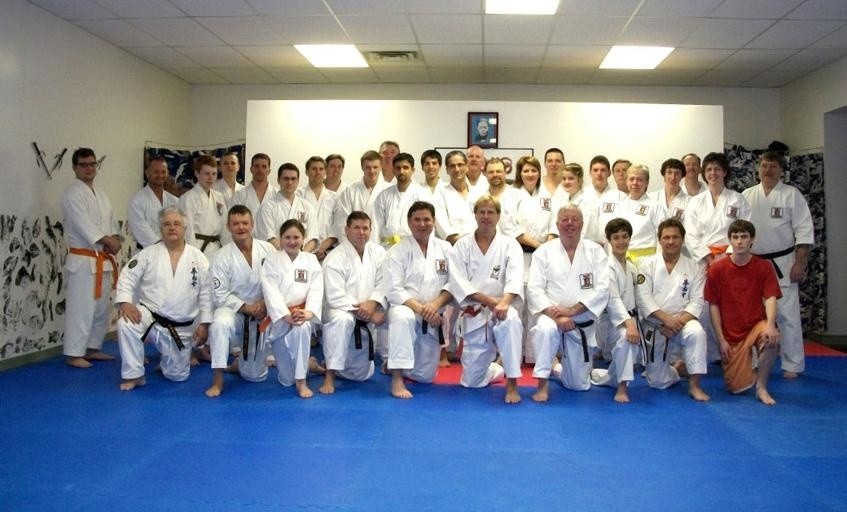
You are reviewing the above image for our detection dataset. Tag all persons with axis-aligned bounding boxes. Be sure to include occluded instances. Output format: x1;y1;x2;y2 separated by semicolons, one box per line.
62;141;125;366
474;118;491;143
113;142;815;405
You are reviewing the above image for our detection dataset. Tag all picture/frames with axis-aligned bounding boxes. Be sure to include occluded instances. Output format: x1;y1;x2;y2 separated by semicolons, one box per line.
466;111;498;148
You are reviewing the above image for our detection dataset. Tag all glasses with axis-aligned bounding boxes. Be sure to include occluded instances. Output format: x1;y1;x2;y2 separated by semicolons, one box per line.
76;161;99;169
